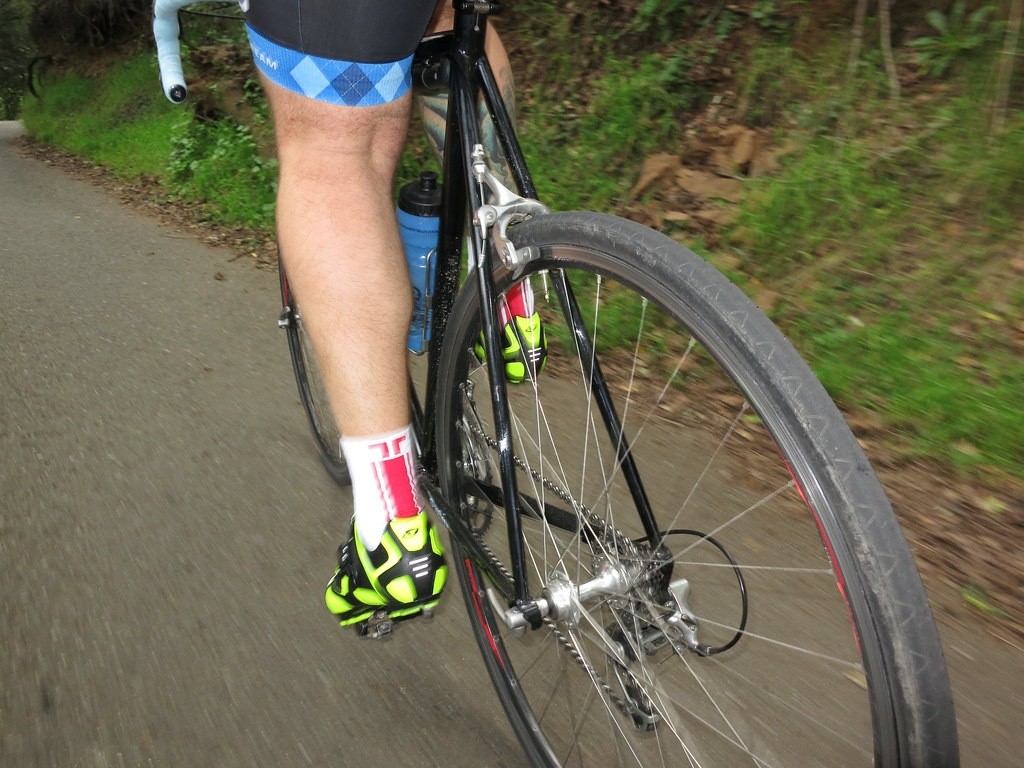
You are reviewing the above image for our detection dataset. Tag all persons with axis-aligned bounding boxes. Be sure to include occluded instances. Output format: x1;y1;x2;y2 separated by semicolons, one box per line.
236;0;548;631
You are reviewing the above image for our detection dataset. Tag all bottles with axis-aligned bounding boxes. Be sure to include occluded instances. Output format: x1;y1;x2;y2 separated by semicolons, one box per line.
386;169;445;350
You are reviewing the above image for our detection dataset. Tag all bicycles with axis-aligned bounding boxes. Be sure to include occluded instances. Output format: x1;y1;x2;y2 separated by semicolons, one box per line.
145;0;964;768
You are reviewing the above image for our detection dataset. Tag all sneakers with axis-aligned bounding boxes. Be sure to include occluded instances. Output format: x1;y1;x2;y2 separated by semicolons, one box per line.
324;512;447;627
471;312;549;384
